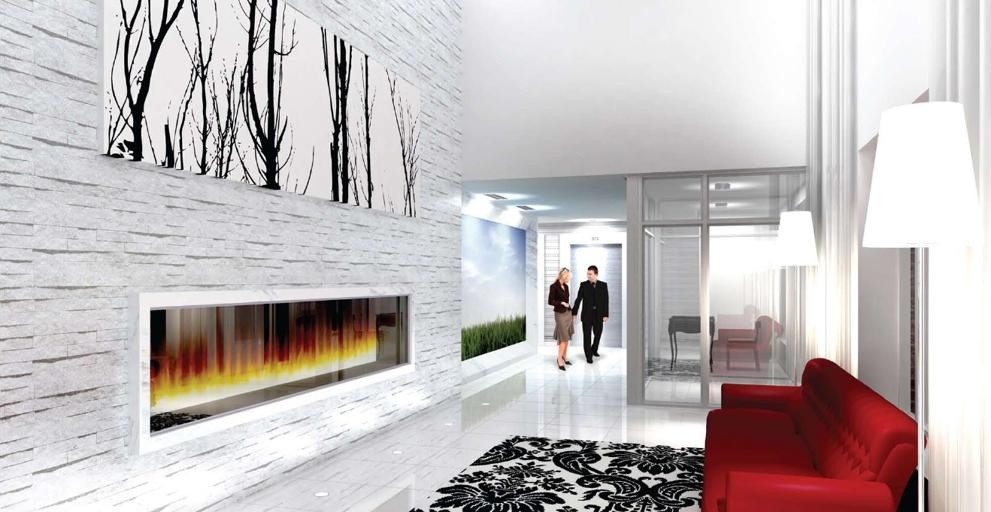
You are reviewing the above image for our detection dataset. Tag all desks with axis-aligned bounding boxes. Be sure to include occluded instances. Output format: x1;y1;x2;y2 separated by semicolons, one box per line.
668;316;715;372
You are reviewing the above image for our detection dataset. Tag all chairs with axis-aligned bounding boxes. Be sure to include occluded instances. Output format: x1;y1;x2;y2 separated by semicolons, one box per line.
726;320;761;371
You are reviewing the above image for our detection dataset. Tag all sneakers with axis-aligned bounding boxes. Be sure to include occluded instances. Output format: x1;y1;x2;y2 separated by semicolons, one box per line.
586;353;599;364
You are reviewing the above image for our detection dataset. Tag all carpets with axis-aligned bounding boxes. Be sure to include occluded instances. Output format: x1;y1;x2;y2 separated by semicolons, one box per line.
409;434;705;512
645;356;705;382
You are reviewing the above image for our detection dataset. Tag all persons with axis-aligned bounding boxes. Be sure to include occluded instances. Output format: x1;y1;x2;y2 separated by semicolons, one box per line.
549;268;576;371
572;265;610;366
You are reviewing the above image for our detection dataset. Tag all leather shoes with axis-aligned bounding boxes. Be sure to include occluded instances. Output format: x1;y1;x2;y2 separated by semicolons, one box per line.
556;356;573;371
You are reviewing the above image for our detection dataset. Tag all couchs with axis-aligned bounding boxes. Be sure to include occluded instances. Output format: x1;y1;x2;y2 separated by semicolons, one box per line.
712;315;784;380
701;357;927;512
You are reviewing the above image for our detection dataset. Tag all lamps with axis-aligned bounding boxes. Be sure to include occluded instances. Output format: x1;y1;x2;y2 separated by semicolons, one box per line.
862;101;982;512
773;210;819;386
